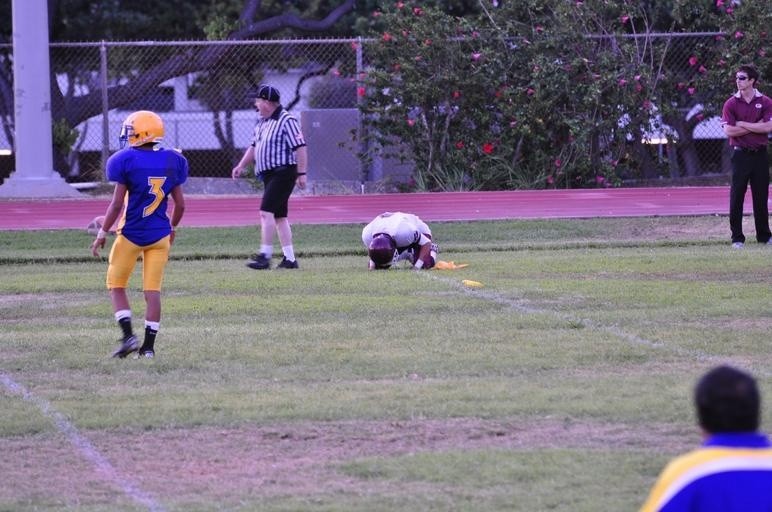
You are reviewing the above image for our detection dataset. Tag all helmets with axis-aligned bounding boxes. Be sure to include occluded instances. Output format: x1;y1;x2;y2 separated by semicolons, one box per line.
368;235;398;266
121;110;165;148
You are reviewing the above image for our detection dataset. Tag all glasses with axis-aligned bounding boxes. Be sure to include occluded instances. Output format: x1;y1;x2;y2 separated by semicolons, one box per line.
735;75;748;81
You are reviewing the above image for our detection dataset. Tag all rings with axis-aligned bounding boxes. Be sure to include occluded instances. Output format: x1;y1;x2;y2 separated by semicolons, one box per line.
300;183;303;186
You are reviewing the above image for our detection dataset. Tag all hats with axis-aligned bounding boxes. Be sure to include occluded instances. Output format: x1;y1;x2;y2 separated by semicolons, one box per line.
248;84;281;101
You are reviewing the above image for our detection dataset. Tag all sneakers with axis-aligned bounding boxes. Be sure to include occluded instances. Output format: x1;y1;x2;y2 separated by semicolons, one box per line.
274;256;298;270
135;351;155;359
112;336;139;359
247;252;272;270
732;242;744;251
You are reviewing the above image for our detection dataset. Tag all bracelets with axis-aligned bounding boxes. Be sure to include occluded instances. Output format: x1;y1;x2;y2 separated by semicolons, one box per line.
96;228;107;239
296;173;306;176
414;258;424;270
170;226;178;231
369;259;375;269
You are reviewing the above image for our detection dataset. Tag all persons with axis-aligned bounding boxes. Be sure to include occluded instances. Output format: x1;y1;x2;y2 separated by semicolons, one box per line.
720;65;772;250
362;211;437;271
638;364;772;511
87;111;187;360
233;85;307;271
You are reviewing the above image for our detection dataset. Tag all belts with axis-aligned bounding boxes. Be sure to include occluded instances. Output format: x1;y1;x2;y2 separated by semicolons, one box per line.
734;145;759;154
